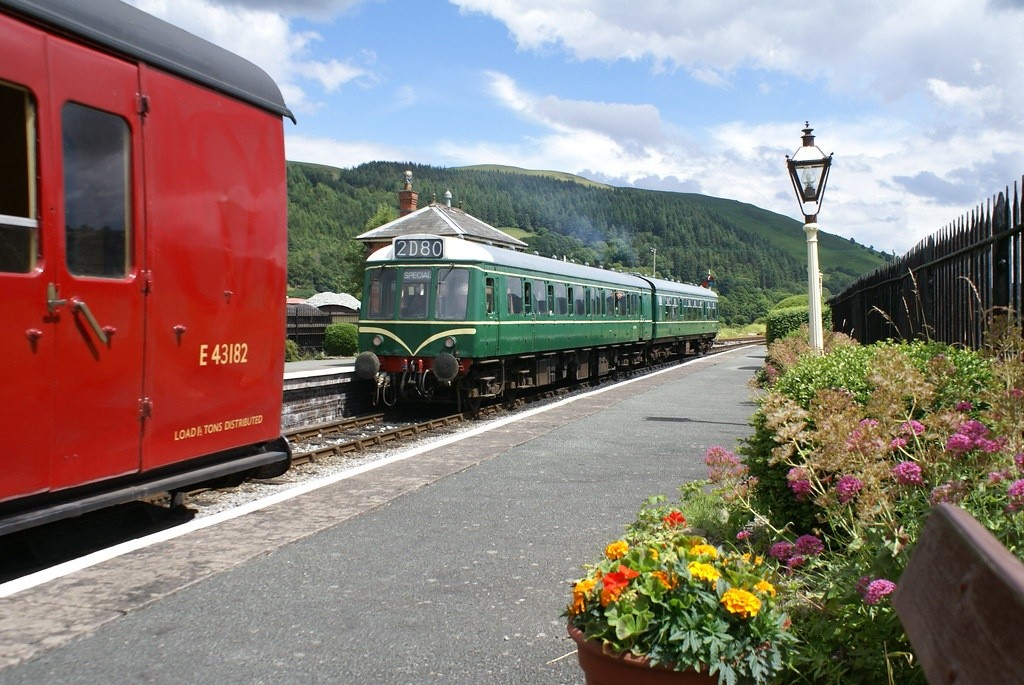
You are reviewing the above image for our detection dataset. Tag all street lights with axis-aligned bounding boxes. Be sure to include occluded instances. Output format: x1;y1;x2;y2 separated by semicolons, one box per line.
784;119;834;357
649;247;656;278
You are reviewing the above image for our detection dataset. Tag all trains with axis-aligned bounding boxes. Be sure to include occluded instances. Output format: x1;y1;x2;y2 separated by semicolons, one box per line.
1;0;296;538
354;232;719;417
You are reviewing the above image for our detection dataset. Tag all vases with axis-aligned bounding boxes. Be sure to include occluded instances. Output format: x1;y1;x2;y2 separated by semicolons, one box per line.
567;616;772;685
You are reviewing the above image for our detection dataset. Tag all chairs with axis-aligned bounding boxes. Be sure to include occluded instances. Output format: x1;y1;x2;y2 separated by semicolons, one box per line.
401;294;426;317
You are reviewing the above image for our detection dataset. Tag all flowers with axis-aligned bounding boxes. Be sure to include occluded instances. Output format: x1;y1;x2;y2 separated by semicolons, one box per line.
704;368;1024;606
559;508;809;685
